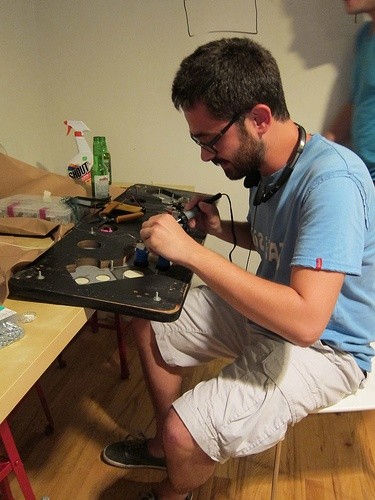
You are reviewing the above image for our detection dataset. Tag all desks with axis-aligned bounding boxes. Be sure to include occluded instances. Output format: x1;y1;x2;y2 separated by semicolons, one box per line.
1;172;195;500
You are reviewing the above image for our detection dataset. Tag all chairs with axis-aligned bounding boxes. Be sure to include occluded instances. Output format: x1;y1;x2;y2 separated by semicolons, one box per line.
269;342;375;500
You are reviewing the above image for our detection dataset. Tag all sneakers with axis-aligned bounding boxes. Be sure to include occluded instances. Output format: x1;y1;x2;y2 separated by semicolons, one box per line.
137;488;193;500
102;432;167;470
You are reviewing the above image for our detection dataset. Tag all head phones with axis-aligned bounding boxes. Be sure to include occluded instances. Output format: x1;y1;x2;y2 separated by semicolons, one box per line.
243;122;306;206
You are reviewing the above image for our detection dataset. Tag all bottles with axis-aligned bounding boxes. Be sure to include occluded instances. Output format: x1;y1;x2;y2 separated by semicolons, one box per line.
91;136;112;199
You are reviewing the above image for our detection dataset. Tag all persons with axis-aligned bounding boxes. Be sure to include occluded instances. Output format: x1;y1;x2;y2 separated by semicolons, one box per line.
100;37;375;500
320;0;375;188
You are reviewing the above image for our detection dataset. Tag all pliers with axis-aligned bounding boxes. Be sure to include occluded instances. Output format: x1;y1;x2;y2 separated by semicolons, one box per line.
90;201;147;230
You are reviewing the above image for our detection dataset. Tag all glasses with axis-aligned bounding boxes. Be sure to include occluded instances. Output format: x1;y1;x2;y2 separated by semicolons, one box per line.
190;111;241;154
